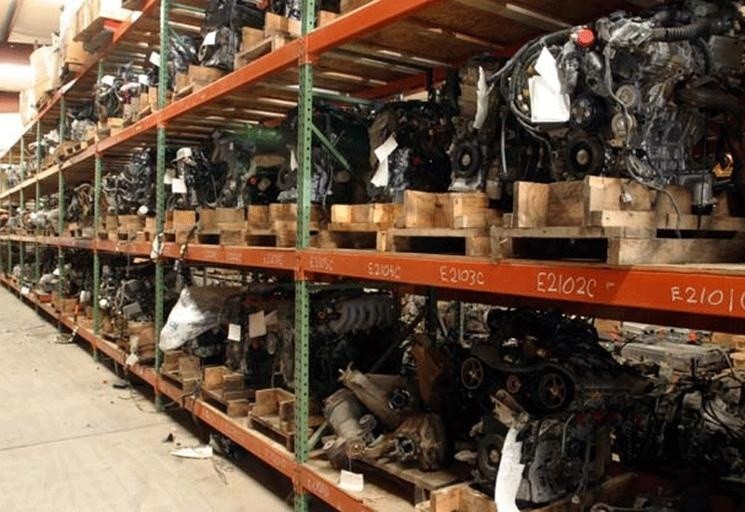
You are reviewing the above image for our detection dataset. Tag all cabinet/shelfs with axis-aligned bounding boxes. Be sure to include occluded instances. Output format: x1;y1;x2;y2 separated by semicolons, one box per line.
0;0;745;512
